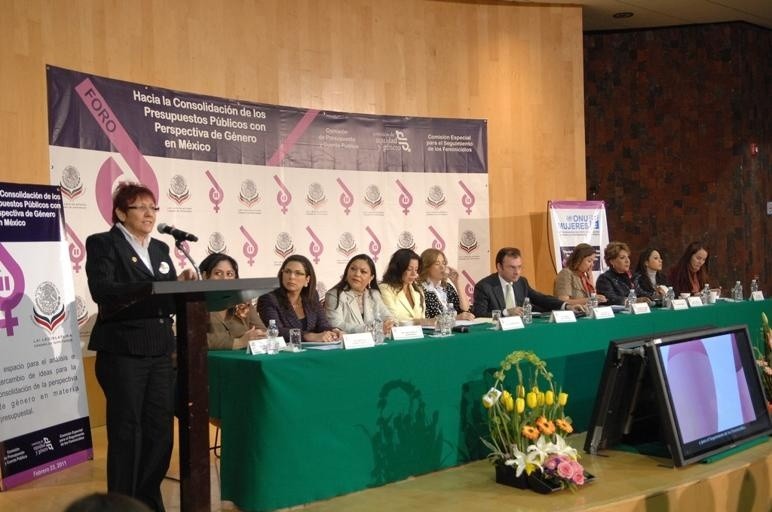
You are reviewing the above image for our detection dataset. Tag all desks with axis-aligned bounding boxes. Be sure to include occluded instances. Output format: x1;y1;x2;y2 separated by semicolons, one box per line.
189;295;772;510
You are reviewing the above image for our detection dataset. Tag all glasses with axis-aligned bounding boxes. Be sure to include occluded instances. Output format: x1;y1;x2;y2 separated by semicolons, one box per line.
127;203;158;212
505;283;515;310
279;268;310;277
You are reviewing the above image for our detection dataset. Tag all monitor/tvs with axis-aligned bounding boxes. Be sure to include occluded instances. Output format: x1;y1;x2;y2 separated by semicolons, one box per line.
579;322;719;457
648;322;768;470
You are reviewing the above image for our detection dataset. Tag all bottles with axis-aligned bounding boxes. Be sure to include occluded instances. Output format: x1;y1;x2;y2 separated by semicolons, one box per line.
267;319;280;355
522;297;533;325
751;279;758;293
589;293;599;318
703;283;710;305
373;312;384;343
440;302;458;335
628;289;638;313
667;286;675;309
735;281;744;302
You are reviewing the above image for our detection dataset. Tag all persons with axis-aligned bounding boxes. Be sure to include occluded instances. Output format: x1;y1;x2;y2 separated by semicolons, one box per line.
256;256;342;343
83;184;199;512
550;243;607;307
416;249;475;323
323;253;399;336
473;247;586;319
376;247;442;328
173;251;270;425
669;241;729;300
594;241;669;308
634;248;681;309
64;490;152;512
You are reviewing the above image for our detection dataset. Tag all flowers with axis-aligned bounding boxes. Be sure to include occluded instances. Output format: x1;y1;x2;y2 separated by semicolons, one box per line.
483;349;590;497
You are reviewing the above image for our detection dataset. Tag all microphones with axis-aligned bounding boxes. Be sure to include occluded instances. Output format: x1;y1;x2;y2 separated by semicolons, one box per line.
156;220;199;244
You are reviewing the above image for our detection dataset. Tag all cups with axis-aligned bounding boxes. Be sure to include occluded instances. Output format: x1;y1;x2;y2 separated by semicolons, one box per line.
399;321;414;326
710;292;719;304
289;328;302;352
491;310;501;326
680;293;691;306
